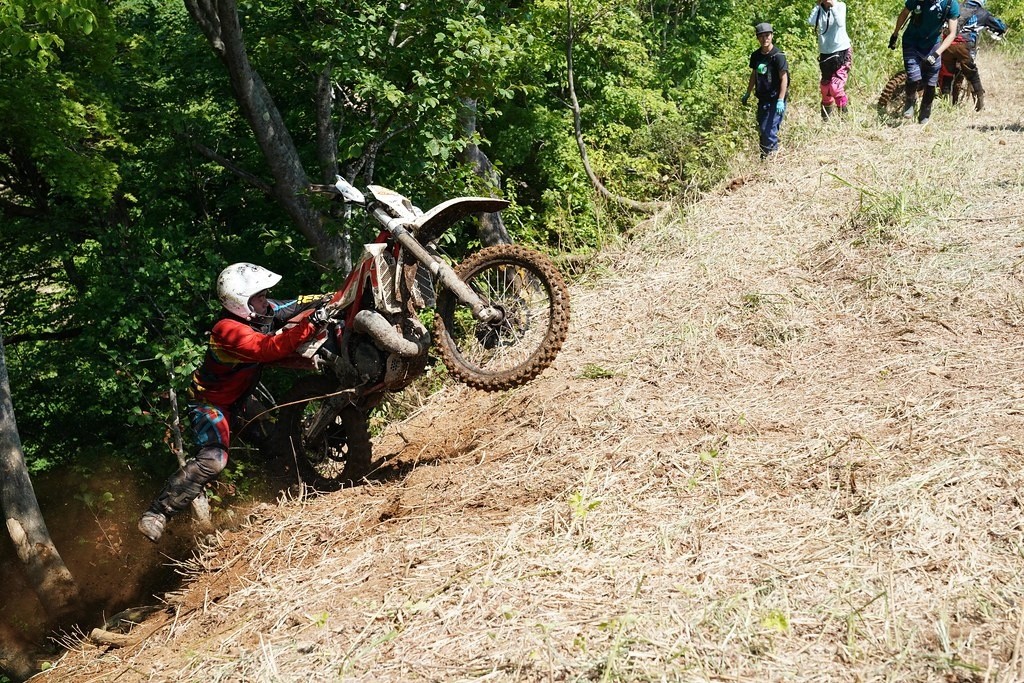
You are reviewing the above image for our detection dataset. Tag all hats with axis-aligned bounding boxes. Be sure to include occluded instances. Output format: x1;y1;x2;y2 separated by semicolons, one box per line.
755;22;773;34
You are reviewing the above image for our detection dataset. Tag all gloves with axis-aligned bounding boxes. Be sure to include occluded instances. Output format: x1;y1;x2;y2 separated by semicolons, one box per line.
308;292;338;309
776;99;785;115
926;50;939;67
306;302;345;336
993;29;1006;36
741;92;750;106
888;34;898;50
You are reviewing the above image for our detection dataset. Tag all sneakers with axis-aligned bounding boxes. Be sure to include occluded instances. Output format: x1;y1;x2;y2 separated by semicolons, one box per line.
137;511;167;541
284;476;302;498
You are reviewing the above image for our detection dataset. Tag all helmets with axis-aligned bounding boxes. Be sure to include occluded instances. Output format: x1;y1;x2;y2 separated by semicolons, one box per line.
970;0;988;9
216;262;283;322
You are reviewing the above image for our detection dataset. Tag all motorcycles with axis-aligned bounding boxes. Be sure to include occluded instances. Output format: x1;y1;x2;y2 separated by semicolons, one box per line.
875;22;1001;119
231;173;571;492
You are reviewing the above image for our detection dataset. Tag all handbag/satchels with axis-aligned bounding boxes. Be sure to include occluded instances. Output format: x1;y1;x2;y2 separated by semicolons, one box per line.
818;50;848;80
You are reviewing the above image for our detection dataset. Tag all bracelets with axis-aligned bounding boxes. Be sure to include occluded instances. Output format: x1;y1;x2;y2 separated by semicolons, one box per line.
932;52;939;59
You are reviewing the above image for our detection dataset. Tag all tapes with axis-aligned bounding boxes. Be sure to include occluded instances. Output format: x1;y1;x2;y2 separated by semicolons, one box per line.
758;63;768;74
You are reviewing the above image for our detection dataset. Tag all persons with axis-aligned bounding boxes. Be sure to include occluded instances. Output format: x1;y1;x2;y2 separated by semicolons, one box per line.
742;23;793;162
133;264;340;542
888;0;1008;126
807;0;852;121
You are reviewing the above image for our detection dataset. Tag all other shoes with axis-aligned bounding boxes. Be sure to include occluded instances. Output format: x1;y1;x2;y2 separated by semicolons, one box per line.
821;105;832;122
941;93;949;103
767;150;777;162
920;117;929;124
903;96;916;119
837;107;850;122
